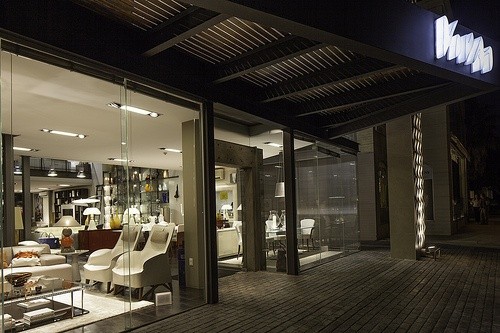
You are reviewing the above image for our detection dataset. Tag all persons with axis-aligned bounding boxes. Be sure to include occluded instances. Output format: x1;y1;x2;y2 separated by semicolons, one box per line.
471;192;488;224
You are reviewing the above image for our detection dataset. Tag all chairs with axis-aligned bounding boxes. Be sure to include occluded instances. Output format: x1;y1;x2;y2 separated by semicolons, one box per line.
111;223;175;300
233;219;315;259
83;224;143;294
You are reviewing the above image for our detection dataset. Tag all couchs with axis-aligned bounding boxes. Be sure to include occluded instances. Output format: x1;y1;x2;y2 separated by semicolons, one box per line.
0;244;71;301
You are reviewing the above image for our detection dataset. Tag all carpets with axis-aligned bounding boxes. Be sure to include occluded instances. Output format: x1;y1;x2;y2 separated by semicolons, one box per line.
0;286;154;333
218;249;344;272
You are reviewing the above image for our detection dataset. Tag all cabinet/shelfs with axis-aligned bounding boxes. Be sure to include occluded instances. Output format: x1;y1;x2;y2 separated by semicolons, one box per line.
103;165;169;223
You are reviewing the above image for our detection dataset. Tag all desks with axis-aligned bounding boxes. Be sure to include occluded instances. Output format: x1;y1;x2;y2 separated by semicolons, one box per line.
55;250;89;282
0;278;90;333
265;229;286;233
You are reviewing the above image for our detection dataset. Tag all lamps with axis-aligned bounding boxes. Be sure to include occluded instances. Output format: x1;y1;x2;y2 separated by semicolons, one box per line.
14;165;22;174
15;207;24;229
274;151;285;197
83;207;101;230
76;171;86;178
54;216;81;253
47;169;57;176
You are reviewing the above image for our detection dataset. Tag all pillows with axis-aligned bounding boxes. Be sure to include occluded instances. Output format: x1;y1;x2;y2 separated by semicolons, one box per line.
8;247;45;268
